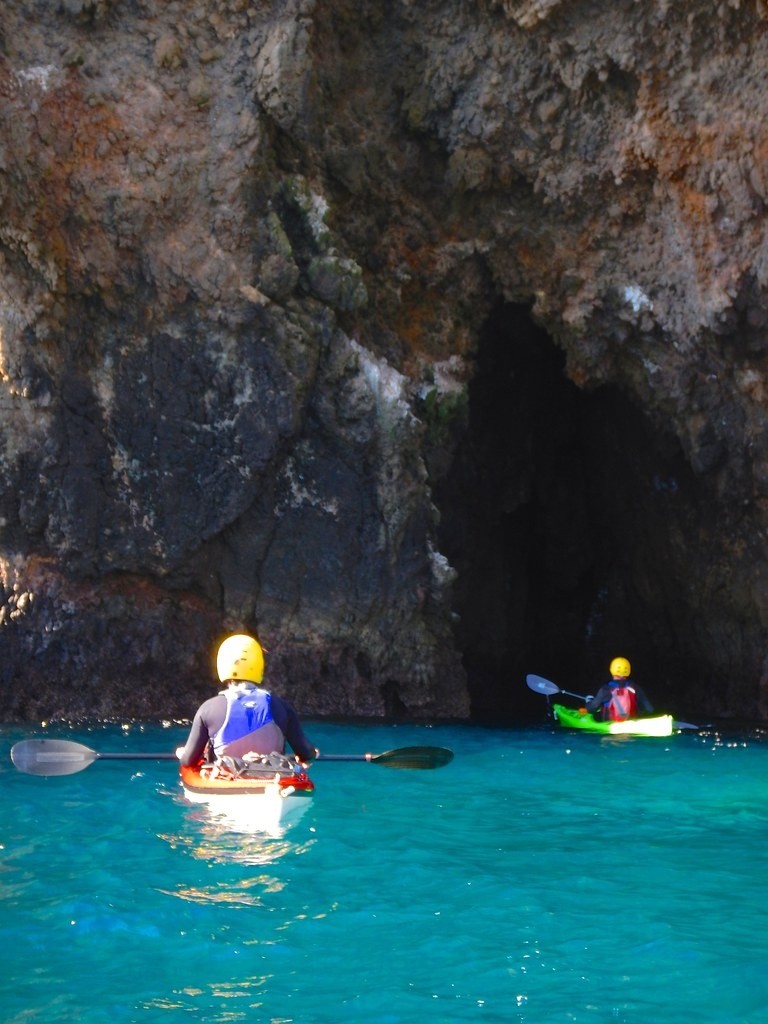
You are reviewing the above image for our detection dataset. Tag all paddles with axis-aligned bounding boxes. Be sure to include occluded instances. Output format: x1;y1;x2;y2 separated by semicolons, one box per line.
528;674;700;730
10;737;455;777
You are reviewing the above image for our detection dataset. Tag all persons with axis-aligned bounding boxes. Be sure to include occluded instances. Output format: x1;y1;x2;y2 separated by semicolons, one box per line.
585;657;653;720
177;634;319;767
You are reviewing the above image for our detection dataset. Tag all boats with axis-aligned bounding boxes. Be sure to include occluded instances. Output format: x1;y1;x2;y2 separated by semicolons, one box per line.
553;701;673;737
177;759;316;831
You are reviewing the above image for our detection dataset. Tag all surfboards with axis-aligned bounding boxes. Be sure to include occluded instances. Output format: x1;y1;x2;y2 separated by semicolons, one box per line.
181;758;314;794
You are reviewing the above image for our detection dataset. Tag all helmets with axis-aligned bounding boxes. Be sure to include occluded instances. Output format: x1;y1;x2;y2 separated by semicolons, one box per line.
609;657;631;677
217;634;265;684
